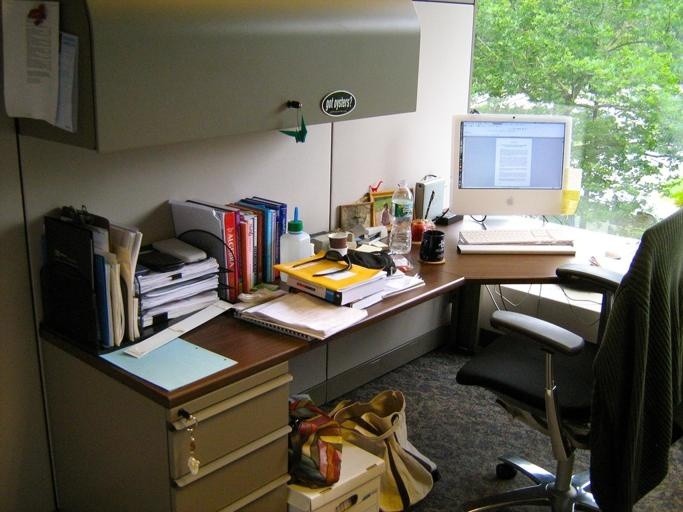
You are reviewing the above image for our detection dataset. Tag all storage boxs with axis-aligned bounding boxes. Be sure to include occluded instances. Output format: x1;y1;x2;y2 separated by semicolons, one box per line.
287;438;386;511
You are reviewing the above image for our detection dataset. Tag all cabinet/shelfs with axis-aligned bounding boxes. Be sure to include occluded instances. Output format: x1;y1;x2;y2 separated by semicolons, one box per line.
40;340;294;512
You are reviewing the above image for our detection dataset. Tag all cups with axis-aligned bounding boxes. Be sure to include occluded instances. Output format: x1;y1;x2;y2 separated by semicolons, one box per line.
419;230;445;261
328;231;347;255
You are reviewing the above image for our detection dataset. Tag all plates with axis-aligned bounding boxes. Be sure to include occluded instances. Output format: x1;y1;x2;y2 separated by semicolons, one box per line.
419;259;446;266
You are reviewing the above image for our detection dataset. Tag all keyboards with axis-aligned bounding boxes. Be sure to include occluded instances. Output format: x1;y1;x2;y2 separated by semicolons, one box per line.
458;225;575;246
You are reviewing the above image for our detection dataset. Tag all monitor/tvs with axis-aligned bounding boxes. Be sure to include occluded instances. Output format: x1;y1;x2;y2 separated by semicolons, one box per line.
450;111;573;228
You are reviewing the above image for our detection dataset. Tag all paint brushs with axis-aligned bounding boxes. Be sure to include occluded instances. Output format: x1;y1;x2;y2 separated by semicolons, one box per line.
424;190;436;220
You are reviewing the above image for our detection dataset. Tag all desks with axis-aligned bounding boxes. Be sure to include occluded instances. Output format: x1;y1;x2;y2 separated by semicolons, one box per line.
38;216;641;410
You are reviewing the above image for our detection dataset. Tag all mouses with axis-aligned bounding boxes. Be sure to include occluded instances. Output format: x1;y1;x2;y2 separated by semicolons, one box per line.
605;248;623;260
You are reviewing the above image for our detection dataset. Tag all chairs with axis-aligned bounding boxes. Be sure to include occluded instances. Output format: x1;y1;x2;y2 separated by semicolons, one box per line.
456;208;681;511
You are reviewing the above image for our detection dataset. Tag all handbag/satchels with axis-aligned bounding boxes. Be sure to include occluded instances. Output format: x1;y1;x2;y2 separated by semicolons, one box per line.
288;388;441;511
347;248;397;276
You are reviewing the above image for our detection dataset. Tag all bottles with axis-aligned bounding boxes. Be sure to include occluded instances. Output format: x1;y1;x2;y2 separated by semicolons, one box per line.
389;179;414;254
280;219;310;284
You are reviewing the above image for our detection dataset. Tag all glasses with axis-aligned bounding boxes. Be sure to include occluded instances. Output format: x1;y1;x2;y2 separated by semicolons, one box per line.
292;250;352;277
